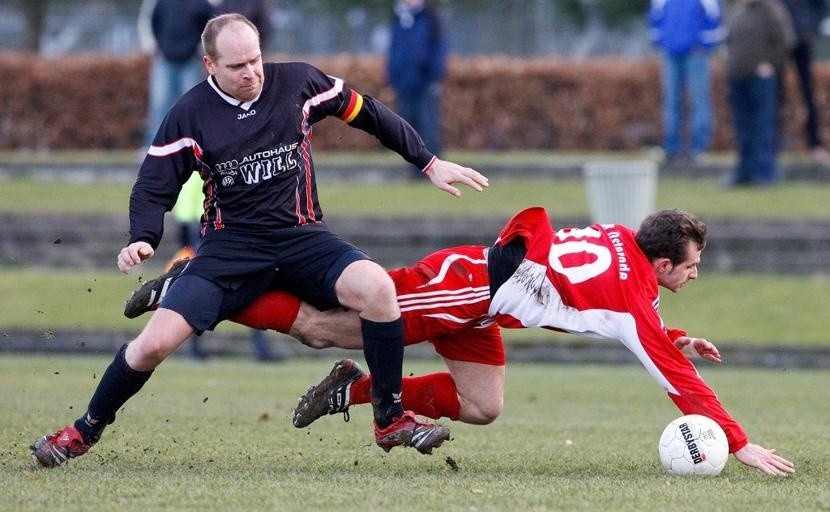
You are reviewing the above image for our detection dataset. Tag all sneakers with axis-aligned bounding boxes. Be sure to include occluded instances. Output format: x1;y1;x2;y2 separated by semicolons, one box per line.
124;256;190;319
292;358;365;429
29;423;95;470
371;407;450;456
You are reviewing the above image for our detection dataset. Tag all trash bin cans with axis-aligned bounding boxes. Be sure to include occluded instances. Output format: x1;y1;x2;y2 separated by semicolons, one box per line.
585;158;658;231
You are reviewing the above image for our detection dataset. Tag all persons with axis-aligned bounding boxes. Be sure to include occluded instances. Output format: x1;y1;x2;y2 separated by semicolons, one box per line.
715;1;797;189
642;1;721;169
26;11;490;467
134;0;213;144
363;0;446;180
119;204;798;480
778;1;830;148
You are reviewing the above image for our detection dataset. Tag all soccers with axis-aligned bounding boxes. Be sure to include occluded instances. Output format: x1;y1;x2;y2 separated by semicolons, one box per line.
660;414;730;479
